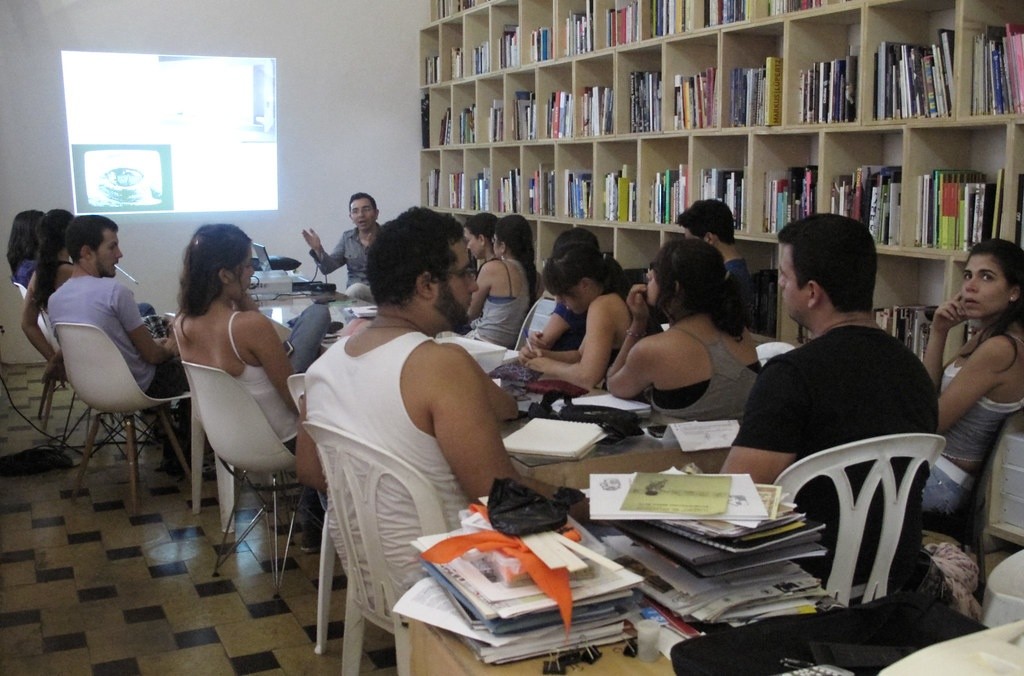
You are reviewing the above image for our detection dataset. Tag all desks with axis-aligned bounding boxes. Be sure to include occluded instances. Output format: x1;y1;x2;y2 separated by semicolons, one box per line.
250;271;732;490
406;575;844;676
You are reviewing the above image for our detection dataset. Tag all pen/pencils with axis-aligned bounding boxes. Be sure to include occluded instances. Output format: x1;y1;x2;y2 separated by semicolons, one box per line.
780;658;815;671
522;329;533;351
114;264;138;285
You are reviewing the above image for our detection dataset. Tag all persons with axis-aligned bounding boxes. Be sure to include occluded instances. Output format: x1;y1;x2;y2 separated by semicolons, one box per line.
921;238;1023;513
519;241;656;391
174;223;324;553
294;205;558;621
464;210;499;262
675;199;750;325
527;228;600;351
718;213;939;606
6;208;191;473
302;191;383;304
606;238;762;427
457;214;545;350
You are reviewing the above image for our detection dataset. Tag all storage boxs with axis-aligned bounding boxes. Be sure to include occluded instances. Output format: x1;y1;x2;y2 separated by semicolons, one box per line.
434;337;508;388
244;269;292;294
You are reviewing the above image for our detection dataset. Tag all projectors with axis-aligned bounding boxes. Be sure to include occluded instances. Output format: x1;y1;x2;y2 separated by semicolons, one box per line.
244;269;293;295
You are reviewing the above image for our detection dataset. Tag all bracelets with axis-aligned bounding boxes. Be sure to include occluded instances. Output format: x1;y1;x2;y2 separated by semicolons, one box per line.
626;328;647;338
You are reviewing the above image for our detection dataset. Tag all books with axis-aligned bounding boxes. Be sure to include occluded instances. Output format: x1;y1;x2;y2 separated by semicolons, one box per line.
501;417;606;458
581;462;830;637
425;0;1024;360
392;496;647;667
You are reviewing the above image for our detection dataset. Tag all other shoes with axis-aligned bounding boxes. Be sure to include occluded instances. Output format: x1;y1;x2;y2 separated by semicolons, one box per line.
301;529;322;553
166;456;215;477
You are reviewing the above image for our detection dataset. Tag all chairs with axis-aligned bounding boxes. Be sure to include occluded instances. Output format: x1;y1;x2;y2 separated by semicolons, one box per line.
756;341;1024;609
514;271;546;351
10;280;328;603
303;420;450;676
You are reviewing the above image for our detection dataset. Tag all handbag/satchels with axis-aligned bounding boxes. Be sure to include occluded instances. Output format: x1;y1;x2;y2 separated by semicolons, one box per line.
558;401;638;444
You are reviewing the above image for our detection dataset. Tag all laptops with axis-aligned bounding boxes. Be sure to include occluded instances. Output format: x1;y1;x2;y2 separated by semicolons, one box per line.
252;242;310;284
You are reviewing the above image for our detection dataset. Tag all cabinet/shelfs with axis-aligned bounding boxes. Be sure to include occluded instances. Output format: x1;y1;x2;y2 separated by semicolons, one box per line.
419;0;1024;373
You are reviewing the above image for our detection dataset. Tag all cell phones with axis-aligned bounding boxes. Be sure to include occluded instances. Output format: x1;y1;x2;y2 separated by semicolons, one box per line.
647;425;667;438
